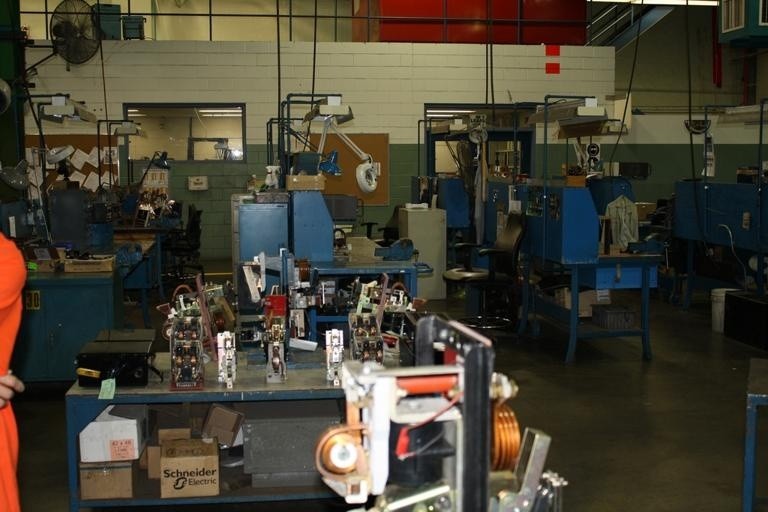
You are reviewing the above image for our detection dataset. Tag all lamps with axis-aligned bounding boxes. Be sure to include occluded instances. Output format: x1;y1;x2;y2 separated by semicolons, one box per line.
122;149;172;214
0;159;38;201
317;114;382;192
266;120;340;176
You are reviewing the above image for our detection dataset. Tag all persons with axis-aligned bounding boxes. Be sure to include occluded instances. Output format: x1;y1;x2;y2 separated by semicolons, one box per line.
0;232;27;512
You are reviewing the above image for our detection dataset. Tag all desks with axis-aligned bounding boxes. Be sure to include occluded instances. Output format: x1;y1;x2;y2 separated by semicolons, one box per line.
65;314;418;509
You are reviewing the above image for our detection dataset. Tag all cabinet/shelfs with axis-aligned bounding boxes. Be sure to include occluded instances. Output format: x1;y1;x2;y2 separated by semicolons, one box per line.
399;208;447;300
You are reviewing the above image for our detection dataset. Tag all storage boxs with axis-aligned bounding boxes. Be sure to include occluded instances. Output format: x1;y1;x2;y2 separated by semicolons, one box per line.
159;438;221;499
554;288;611;318
78;405;149;464
202;402;246;447
243;398;341;490
591;304;638;330
79;460;134;500
636;202;658;222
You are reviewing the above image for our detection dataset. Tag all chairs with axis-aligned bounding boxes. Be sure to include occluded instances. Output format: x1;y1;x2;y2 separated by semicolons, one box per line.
362;204;407;246
441;210;530;342
172;204;197;270
162;209;203;275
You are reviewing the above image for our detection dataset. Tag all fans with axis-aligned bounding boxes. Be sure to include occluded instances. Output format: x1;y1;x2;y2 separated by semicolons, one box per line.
19;0;102;100
